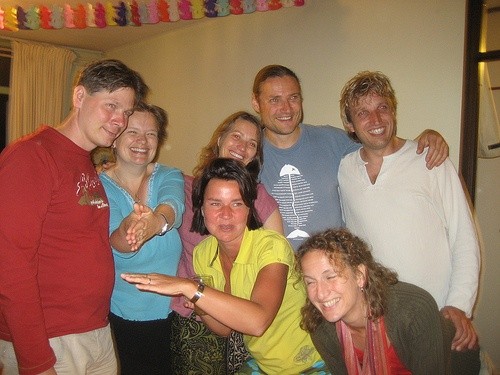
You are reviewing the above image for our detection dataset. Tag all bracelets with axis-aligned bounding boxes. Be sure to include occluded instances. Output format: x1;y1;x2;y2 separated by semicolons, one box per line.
190;284;204;303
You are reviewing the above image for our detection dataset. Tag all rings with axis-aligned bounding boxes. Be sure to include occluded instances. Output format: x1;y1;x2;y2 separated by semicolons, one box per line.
148;278;152;285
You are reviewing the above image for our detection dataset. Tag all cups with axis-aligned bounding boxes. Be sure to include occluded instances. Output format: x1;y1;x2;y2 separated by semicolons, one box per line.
193;275;214;315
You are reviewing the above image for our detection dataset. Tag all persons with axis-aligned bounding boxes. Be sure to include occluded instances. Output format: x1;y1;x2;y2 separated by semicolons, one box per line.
252;64;448;258
95;111;285;375
0;59;151;375
338;70;481;351
98;103;186;375
291;228;500;375
121;158;331;375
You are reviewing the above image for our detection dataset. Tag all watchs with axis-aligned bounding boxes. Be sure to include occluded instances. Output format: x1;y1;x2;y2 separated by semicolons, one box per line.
153;211;170;236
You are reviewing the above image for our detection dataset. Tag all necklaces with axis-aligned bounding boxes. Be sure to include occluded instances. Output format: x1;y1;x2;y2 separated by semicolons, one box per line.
114;168;146;203
